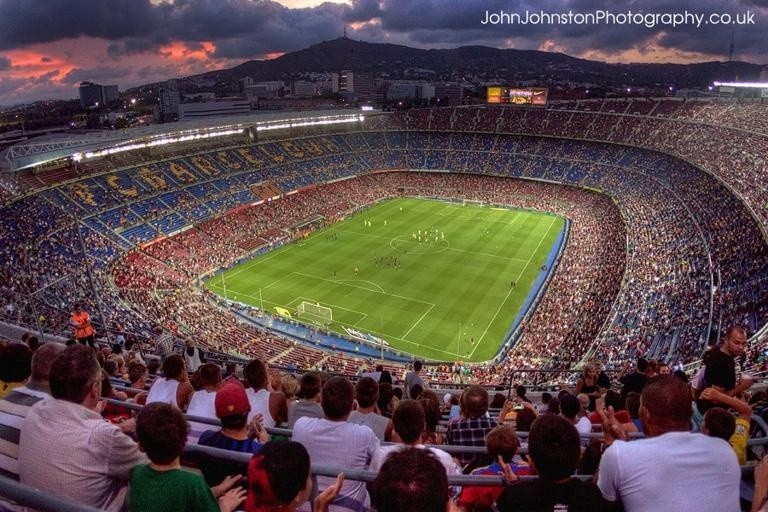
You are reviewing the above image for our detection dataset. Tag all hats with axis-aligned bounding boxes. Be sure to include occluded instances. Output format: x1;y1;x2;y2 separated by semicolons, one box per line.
213;378;251;417
245;440;310;507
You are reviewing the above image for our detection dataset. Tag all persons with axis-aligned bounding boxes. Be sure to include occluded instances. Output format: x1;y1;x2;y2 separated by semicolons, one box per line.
0;98;768;512
